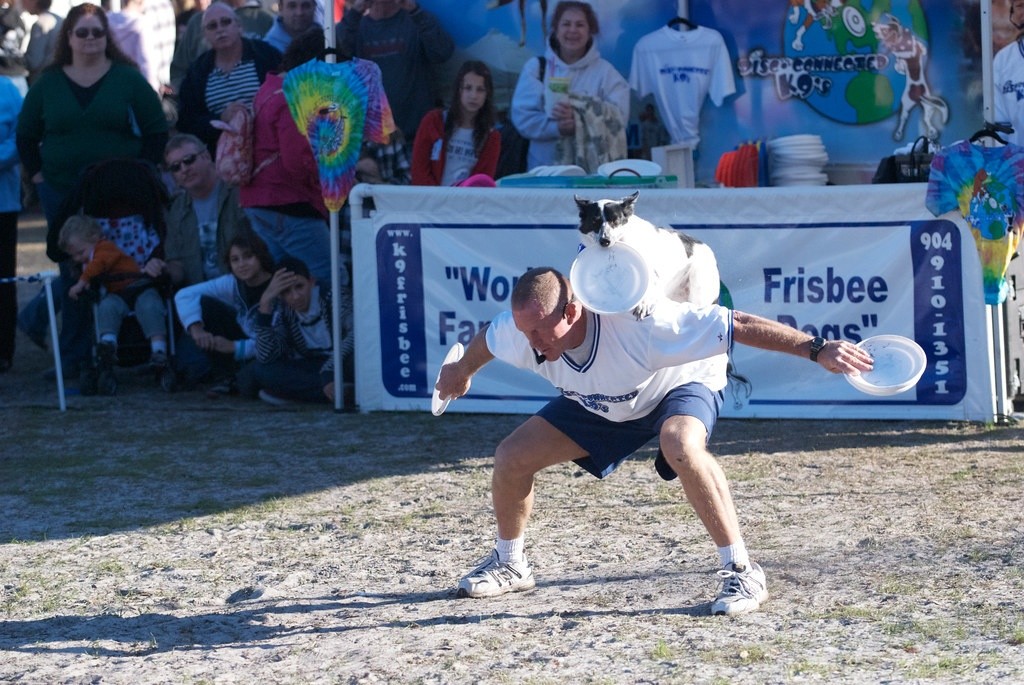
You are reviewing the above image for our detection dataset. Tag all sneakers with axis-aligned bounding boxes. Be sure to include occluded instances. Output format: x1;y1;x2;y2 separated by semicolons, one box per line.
710;560;769;615
456;547;535;600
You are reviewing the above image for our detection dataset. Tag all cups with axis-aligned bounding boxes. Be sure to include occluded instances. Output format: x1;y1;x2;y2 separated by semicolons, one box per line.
548;78;570;108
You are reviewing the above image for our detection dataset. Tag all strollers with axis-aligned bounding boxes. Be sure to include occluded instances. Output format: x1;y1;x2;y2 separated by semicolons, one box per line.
57;154;196;396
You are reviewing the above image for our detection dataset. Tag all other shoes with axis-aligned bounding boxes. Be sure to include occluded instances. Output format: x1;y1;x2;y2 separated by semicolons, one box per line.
259;388;305;411
324;381;358;406
142;350;168;371
93;338;119;363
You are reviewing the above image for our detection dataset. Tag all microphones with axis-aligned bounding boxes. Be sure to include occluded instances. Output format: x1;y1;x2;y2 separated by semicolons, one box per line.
532;347;546;365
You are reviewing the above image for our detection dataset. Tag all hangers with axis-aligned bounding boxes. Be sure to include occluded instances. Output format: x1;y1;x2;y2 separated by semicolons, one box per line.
668;2;698;31
969;107;1007;148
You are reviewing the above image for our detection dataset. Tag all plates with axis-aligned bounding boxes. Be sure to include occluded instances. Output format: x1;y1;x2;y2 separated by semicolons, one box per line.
844;333;925;394
530;158;662;177
568;242;647;314
716;131;831;187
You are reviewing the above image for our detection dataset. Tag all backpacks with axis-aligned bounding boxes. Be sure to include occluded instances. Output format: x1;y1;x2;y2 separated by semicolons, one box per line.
210;104;280;188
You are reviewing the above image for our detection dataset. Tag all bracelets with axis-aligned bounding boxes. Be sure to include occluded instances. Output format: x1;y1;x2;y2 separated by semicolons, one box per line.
409;4;419;14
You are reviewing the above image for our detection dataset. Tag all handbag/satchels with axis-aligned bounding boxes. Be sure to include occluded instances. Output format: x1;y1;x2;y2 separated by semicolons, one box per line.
870;135;934;183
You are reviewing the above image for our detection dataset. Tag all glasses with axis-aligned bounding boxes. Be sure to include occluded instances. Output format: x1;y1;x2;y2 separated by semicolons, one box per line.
204;14;237;34
74;26;105;39
170;147;207;172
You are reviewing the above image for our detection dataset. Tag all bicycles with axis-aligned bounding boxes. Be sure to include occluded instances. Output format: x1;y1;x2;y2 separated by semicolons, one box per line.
864;118;1020;305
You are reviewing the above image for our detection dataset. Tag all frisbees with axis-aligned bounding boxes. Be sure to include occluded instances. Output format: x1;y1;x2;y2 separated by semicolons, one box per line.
714;131;832;187
450;157;662;188
842;333;928;398
431;342;465;416
568;240;650;316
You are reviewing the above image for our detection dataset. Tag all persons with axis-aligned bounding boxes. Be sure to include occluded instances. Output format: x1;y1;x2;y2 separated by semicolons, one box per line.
0;75;25;372
411;61;502;188
510;1;631;174
263;0;323;55
170;0;273;89
175;2;283;164
238;28;349;294
162;132;244;286
246;254;355;406
16;3;169;264
58;215;171;369
0;0;176;92
991;0;1024;149
337;0;453;122
159;233;282;398
435;268;875;614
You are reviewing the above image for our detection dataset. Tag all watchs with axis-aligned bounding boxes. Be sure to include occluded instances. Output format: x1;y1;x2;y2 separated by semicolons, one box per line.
809;337;826;362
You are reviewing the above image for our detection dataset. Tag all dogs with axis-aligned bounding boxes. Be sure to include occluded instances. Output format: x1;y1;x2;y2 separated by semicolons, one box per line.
574;191;721;321
